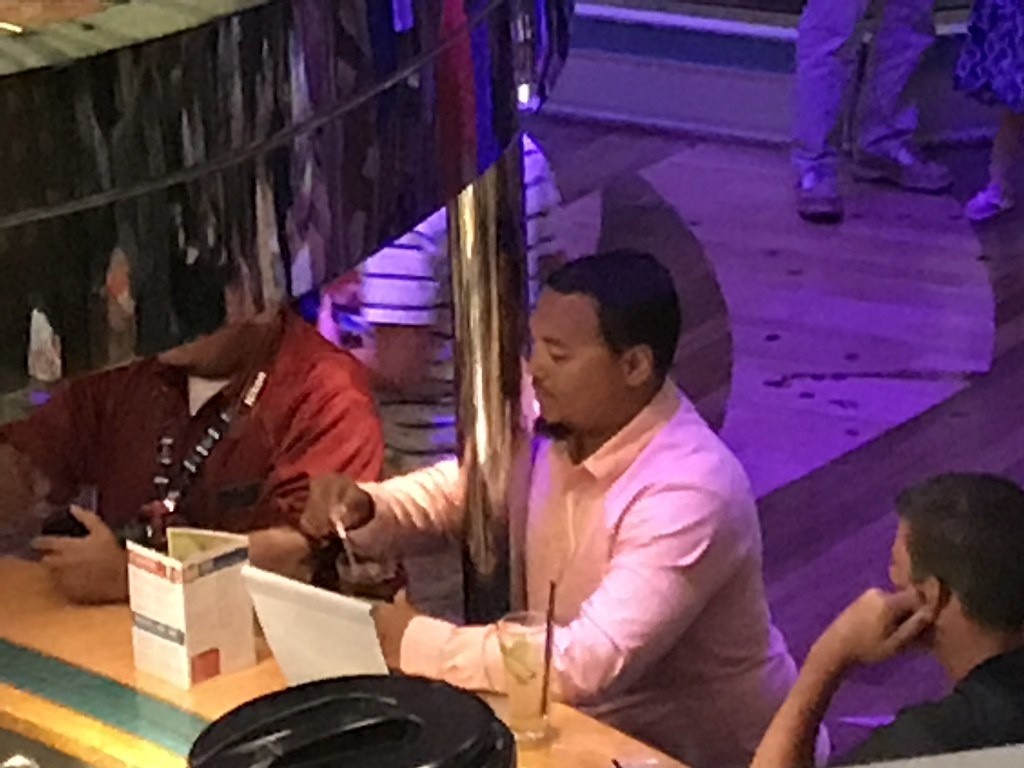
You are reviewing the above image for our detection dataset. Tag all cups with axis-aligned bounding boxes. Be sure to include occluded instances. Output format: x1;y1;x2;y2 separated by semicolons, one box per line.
494;610;554;750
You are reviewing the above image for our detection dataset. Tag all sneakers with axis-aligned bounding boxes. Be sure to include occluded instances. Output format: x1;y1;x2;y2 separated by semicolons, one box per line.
852;143;954;195
793;171;843;225
965;179;1016;221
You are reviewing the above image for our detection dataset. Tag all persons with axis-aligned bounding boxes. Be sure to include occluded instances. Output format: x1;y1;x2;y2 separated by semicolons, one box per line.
325;131;562;470
748;471;1022;768
0;301;409;607
792;0;958;217
299;248;833;768
948;0;1024;219
